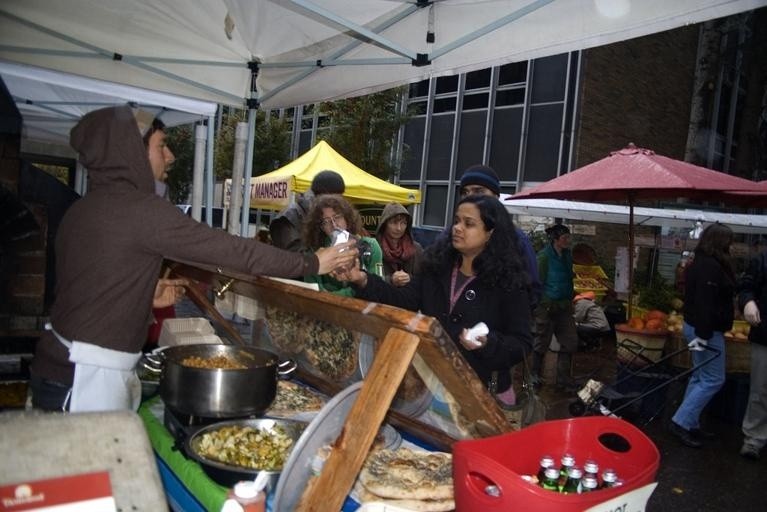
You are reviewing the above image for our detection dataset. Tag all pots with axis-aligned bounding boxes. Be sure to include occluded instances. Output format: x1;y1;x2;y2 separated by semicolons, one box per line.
140;342;298;426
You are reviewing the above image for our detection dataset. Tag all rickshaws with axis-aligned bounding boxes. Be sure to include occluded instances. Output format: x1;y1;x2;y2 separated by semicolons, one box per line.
568;337;722;449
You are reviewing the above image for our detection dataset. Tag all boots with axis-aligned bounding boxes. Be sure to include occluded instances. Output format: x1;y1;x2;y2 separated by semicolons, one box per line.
532;351;544;389
556;352;582;393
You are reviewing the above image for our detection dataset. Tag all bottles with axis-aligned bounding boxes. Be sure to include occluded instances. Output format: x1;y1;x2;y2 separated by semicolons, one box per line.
537;452;623;494
226;470;268;512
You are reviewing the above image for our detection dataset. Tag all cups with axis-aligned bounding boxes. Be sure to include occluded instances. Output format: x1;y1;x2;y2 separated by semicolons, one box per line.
328;226;371;273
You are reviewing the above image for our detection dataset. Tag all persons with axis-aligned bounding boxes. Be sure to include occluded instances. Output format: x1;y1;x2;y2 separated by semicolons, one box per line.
27;105;359;415
253;172;424;297
531;224;612;416
670;220;767;457
329;165;537;406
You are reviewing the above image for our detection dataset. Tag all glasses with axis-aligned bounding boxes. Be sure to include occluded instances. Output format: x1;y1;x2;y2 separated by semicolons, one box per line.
322;215;342;225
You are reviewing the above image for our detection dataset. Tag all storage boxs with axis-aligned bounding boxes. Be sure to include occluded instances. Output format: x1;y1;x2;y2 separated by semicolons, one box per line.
449;418;662;512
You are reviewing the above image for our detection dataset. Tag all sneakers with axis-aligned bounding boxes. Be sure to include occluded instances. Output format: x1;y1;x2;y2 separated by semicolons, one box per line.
669;419;702;448
740;442;759;459
696;429;716;441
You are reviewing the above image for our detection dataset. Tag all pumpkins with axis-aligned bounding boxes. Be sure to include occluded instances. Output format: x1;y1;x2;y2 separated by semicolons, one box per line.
628;310;666;331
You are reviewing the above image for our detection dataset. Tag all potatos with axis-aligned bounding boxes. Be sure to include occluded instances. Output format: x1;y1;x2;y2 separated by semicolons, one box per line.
724;326;750;339
668;314;682;331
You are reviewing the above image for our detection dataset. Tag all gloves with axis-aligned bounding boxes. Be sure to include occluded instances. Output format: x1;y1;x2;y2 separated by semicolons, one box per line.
687;337;708;352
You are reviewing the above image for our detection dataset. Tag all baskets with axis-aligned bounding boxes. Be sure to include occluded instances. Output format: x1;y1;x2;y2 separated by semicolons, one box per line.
614;326;666;361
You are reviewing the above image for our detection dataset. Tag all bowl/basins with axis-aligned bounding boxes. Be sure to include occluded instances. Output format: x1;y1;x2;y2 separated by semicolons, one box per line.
184;416;310;489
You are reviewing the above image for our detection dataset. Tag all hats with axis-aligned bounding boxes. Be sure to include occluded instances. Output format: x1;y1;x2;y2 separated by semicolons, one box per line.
131;107;155;138
461;164;500;196
311;171;345;194
